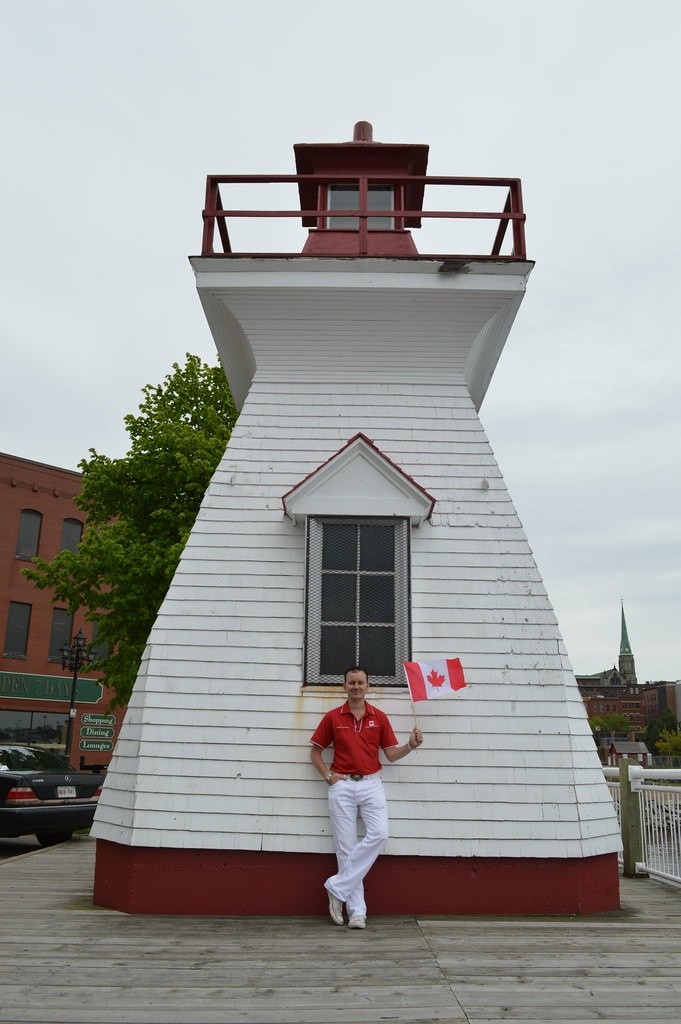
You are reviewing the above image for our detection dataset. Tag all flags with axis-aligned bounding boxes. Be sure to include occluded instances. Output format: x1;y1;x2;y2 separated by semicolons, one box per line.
404;658;466;702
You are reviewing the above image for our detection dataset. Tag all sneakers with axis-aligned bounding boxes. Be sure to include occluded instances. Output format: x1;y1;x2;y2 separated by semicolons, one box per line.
348;916;367;930
326;891;345;925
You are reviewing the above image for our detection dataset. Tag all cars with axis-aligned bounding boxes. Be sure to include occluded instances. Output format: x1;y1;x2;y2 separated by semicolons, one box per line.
0;744;106;847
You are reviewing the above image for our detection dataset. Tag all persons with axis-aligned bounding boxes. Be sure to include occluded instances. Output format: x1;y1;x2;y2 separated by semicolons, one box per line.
309;667;424;929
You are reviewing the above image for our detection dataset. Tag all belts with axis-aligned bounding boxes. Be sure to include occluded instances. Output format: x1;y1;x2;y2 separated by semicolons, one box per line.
340;772;381;781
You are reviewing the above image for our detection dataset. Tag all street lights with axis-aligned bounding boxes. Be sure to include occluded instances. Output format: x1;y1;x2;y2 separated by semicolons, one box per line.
59;628;97;764
44;716;46;728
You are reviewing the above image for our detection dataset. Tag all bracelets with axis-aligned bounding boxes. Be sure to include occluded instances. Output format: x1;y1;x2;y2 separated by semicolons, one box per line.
407;741;414;751
324;774;332;783
